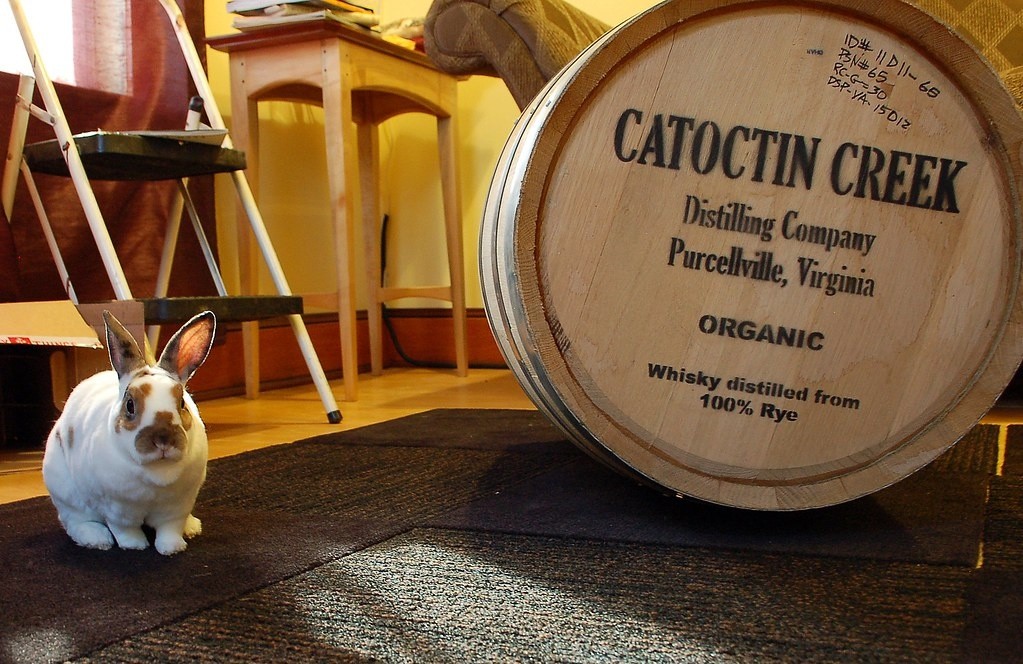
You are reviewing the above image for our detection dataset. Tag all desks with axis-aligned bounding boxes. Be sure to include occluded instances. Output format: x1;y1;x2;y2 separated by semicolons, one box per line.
206;19;468;402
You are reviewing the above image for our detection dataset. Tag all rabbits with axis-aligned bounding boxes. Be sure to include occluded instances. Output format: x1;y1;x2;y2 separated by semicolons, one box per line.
41;310;217;555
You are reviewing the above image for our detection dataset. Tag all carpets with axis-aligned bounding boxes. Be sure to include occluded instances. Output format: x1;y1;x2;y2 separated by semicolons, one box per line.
0;407;1023;664
